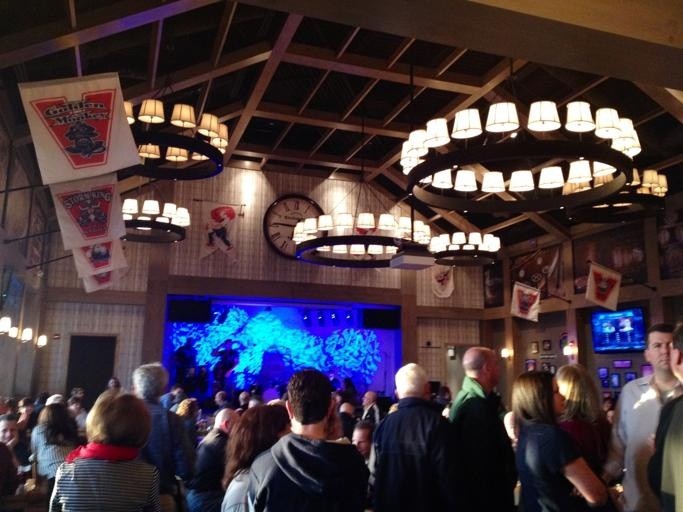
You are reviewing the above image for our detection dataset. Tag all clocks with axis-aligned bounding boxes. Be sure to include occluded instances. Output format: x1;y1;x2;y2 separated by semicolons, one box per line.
264;193;329;261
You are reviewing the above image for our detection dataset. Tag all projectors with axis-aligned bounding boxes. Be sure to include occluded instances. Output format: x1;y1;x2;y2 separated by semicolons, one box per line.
391;241;437;270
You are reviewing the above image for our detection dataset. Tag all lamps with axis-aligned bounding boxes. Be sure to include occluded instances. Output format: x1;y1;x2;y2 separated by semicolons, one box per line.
122;180;190;243
292;107;432;271
125;68;228;180
429;194;501;267
561;159;668;224
397;57;642;212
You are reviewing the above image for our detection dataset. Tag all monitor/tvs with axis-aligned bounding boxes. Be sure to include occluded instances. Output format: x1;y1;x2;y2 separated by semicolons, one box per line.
588;306;649;354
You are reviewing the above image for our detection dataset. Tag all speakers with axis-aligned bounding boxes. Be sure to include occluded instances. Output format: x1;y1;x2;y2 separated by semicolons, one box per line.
168;298;210;322
363;308;401;329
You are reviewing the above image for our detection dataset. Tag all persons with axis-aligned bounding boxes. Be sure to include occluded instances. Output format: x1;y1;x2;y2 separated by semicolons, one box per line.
355;391;380;424
213;391;231;417
175;337;198;385
17;398;38;433
160;385;183;409
447;346;522;512
45;394;66;406
130;363;193;512
350;421;375;458
0;413;32;481
436;386;452;406
210;339;246;392
335;391;348;404
338;402;357;441
176;398;197;441
510;370;607;512
235;391;249;416
570;324;680;512
371;362;453;512
67;397;87;432
27;404;78;499
245;368;370;512
48;388;162;512
186;409;238;512
70;387;84;399
504;365;611;474
645;325;683;512
108;376;128;394
220;403;291;512
